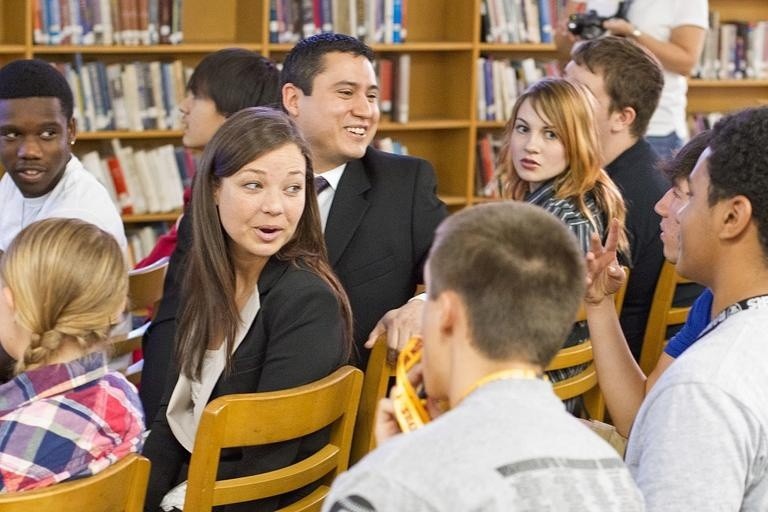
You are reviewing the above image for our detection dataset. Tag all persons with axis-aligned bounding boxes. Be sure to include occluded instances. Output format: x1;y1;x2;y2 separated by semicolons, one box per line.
582;129;716;440
137;107;356;512
121;41;287;277
276;31;452;369
491;77;628;417
1;55;135;277
584;2;709;163
558;36;673;368
0;216;152;498
322;198;644;512
624;103;768;512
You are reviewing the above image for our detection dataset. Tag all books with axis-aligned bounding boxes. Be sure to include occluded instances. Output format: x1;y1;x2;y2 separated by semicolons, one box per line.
30;0;205;266
477;2;584;195
270;0;411;157
683;19;767;134
23;1;411;269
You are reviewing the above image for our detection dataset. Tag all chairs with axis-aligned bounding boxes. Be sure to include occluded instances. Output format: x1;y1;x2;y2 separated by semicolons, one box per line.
347;325;452;467
1;451;155;511
174;362;365;512
543;264;629;422
639;249;706;387
99;256;172;390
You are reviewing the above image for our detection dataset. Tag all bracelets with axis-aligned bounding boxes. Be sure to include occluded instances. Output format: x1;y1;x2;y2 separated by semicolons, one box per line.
628;27;642;41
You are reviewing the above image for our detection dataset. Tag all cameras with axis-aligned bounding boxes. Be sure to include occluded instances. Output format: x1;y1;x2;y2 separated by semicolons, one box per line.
567;10;609;39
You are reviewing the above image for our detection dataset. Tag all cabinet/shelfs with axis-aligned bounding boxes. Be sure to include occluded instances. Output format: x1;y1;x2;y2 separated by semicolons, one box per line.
0;0;767;234
